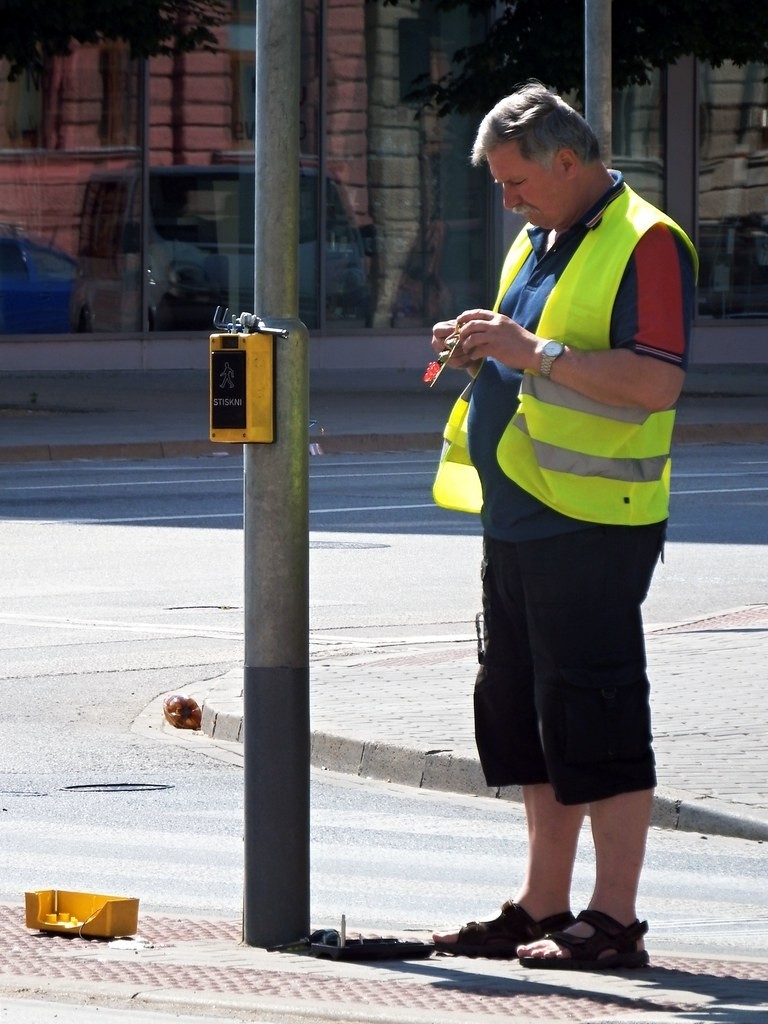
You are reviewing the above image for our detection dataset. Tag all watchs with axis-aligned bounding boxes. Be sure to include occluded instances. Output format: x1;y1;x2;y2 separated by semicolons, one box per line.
541;340;564;379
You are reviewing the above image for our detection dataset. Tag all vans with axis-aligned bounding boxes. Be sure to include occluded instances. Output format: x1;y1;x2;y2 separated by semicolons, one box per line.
78;165;371;333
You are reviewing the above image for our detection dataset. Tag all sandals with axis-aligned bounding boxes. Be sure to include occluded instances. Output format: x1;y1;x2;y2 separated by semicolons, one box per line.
520;909;650;969
434;899;575;957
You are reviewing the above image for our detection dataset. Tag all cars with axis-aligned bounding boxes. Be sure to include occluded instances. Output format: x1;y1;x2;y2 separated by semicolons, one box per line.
698;220;768;319
0;236;77;334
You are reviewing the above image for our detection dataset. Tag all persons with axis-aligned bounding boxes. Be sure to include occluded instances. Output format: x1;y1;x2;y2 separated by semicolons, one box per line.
428;86;699;969
